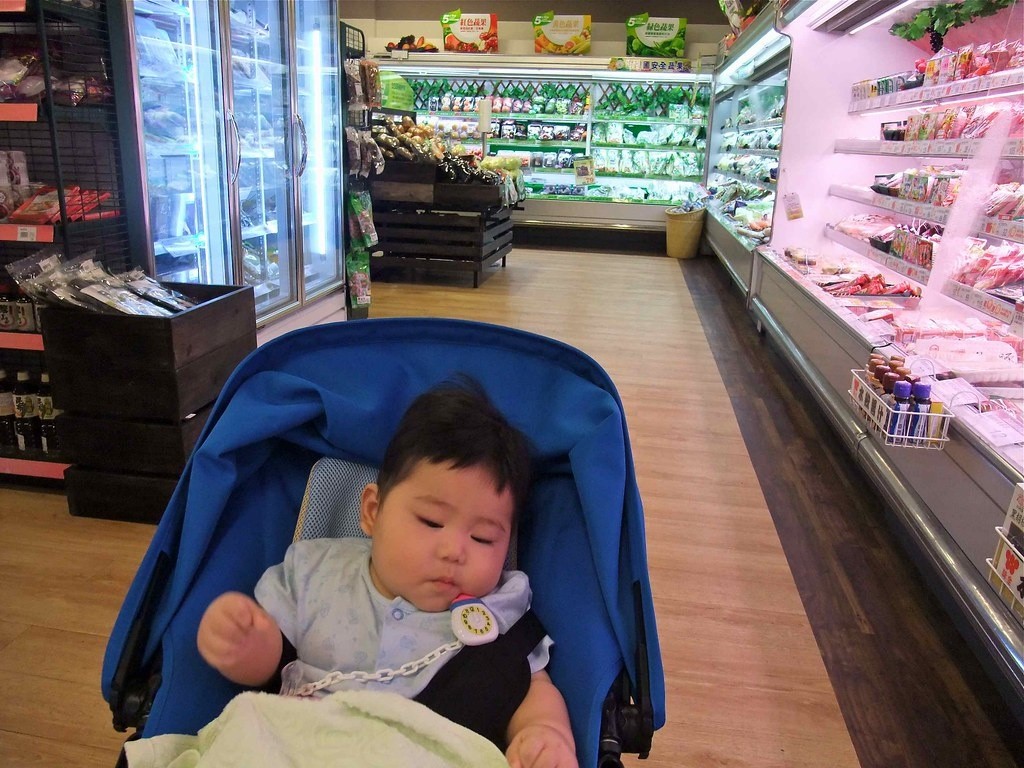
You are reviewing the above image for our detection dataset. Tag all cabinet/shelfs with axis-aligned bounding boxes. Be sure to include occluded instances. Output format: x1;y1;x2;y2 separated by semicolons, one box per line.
0;0;157;482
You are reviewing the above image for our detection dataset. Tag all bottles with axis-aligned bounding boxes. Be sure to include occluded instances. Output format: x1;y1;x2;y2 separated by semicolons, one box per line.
0;283;48;334
0;369;59;455
864;353;932;444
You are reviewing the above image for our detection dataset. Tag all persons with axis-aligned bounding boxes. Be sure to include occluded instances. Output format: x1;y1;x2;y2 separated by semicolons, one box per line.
198;380;578;768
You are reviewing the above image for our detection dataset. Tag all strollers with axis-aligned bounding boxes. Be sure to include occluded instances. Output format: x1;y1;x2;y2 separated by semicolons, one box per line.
99;318;668;768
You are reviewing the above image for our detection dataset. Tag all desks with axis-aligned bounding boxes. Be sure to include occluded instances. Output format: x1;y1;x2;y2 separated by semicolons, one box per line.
368;179;520;288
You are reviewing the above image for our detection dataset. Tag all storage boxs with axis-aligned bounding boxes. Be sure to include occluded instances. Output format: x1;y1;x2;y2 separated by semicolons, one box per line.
39;277;256;525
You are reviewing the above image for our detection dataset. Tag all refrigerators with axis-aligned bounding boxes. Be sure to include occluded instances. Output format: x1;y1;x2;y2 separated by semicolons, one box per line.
108;1;348;347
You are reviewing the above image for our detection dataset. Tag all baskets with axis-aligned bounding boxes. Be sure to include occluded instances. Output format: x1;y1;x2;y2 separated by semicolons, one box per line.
847;367;954;450
982;524;1024;619
